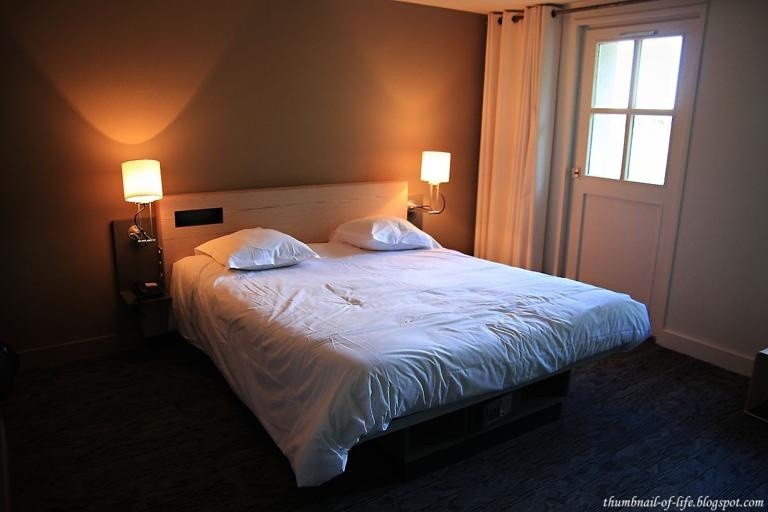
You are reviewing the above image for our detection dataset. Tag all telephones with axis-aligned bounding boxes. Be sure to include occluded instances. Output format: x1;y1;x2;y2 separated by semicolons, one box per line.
132;281;164;299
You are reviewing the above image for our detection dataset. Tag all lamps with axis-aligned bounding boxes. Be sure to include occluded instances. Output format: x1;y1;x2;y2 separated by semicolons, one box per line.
122;160;163;247
408;151;452;218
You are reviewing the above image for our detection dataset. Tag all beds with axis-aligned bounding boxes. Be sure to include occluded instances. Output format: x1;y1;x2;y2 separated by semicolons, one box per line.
155;180;652;487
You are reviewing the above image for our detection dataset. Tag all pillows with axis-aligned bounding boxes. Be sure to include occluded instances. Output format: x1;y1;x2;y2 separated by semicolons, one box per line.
194;226;321;271
328;216;446;250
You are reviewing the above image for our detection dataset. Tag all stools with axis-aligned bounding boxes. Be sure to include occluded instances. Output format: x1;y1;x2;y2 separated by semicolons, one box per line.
744;348;768;423
